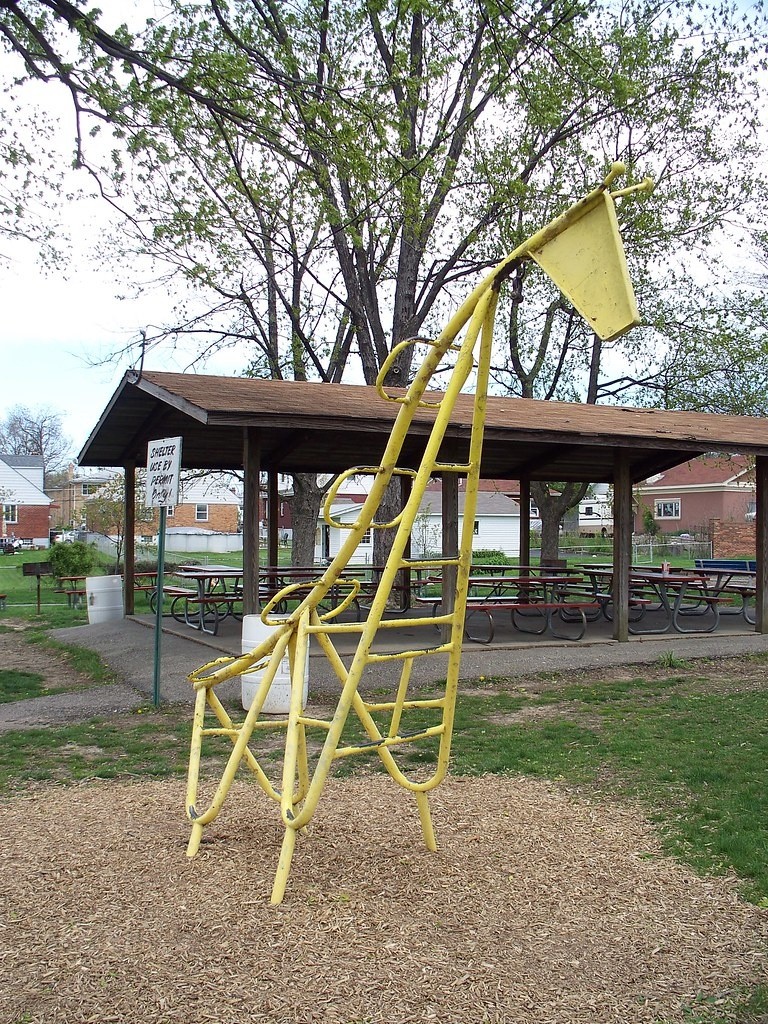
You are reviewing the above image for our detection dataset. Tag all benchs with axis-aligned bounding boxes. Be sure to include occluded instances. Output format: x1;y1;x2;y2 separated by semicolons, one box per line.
121;573;758;611
53;548;766;652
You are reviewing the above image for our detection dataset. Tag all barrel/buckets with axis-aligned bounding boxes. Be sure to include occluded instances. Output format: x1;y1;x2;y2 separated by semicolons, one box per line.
241;613;310;713
87;575;123;625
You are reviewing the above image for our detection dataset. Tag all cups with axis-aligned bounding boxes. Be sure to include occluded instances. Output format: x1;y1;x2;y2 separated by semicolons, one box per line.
661;562;670;577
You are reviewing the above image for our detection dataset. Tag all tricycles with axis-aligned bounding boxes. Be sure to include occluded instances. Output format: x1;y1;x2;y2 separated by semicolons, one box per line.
2;541;15;555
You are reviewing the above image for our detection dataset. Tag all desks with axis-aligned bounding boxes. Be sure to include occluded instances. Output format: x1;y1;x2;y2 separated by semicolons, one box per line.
57;574;86;609
575;563;682;609
170;569;365;636
119;571;173;597
176;564;244;616
580;568;711;633
470;564;592;623
428;576;587;645
258;562;410;614
684;568;757;624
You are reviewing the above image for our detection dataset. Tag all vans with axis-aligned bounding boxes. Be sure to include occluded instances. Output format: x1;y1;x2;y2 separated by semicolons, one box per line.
580;500;615;538
50;531;63;545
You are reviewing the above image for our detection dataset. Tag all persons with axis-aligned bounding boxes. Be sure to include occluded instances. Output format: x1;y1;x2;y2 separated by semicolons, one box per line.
601;528;607;546
3;536;24;553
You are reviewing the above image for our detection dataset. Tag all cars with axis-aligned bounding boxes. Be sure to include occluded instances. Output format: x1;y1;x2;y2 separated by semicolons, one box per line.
22;542;37;551
56;531;74;544
